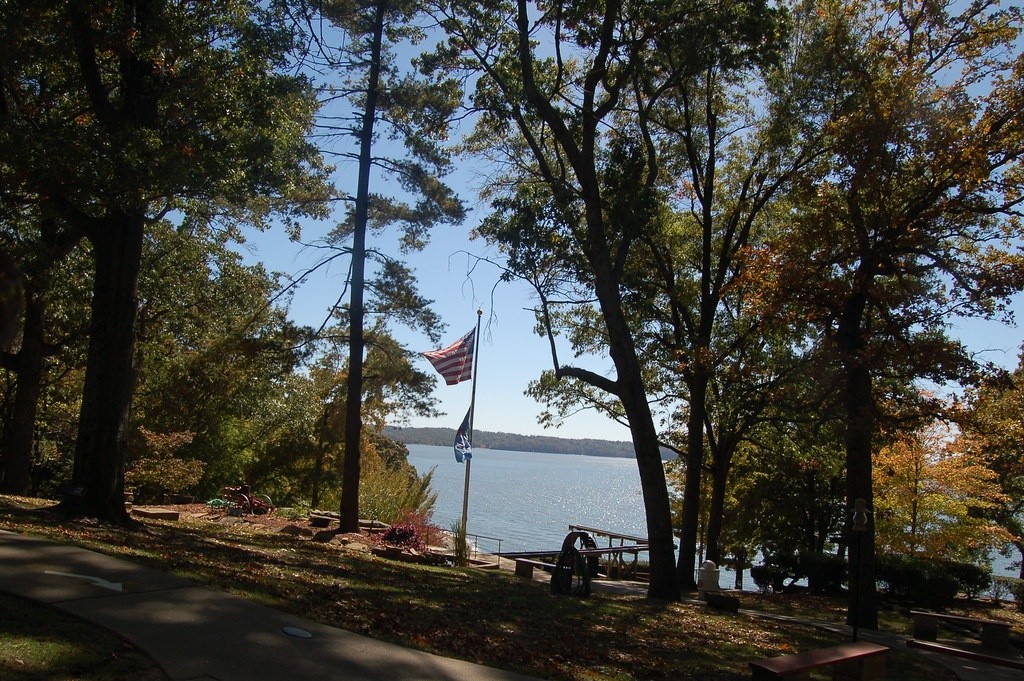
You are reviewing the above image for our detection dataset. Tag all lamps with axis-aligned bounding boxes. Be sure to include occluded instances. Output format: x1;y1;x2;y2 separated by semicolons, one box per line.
851;499;871;532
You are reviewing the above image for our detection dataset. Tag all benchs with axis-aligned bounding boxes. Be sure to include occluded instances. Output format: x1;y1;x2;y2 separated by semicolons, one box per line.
747;640;890;681
514;557;571;586
910;610;1013;651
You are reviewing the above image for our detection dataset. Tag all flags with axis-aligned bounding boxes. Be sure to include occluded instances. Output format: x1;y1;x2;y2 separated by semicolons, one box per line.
454;407;473;462
423;326;476;385
